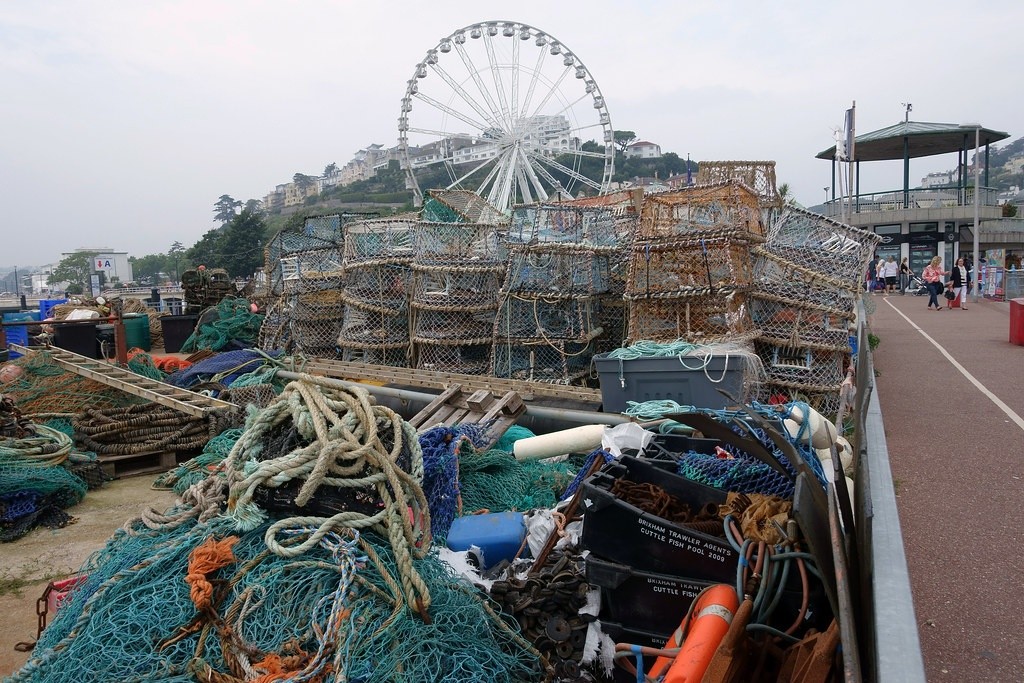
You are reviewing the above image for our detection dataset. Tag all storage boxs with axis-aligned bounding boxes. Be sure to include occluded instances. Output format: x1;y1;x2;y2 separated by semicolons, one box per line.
277;157;883;671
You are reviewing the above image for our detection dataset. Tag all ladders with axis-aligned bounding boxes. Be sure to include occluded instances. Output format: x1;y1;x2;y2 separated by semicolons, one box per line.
8;341;244;419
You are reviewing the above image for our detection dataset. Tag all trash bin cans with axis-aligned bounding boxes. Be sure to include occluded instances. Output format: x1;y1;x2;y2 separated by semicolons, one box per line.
1006;298;1024;346
157;315;198;354
54;323;98;361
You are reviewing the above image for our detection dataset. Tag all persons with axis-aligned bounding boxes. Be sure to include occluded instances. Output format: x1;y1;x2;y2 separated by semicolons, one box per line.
864;255;915;296
948;257;971;310
921;256;949;311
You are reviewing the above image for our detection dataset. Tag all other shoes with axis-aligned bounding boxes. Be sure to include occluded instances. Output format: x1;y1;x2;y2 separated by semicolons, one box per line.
949;304;952;309
928;307;932;310
937;306;942;311
962;307;968;310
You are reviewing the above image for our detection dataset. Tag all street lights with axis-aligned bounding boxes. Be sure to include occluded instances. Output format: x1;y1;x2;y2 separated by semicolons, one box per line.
554;181;563;201
257;239;262;266
13;265;19;298
823;186;830;217
174;256;179;283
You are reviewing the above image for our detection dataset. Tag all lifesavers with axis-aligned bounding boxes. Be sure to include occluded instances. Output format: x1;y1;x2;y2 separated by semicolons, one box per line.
645;582;739;683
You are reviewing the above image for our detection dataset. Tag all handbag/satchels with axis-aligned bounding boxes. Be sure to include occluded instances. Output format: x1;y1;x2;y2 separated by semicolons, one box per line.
937;282;944;294
944;290;955;300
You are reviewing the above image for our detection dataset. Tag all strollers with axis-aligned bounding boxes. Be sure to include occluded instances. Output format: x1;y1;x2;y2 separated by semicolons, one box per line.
907;273;929;297
